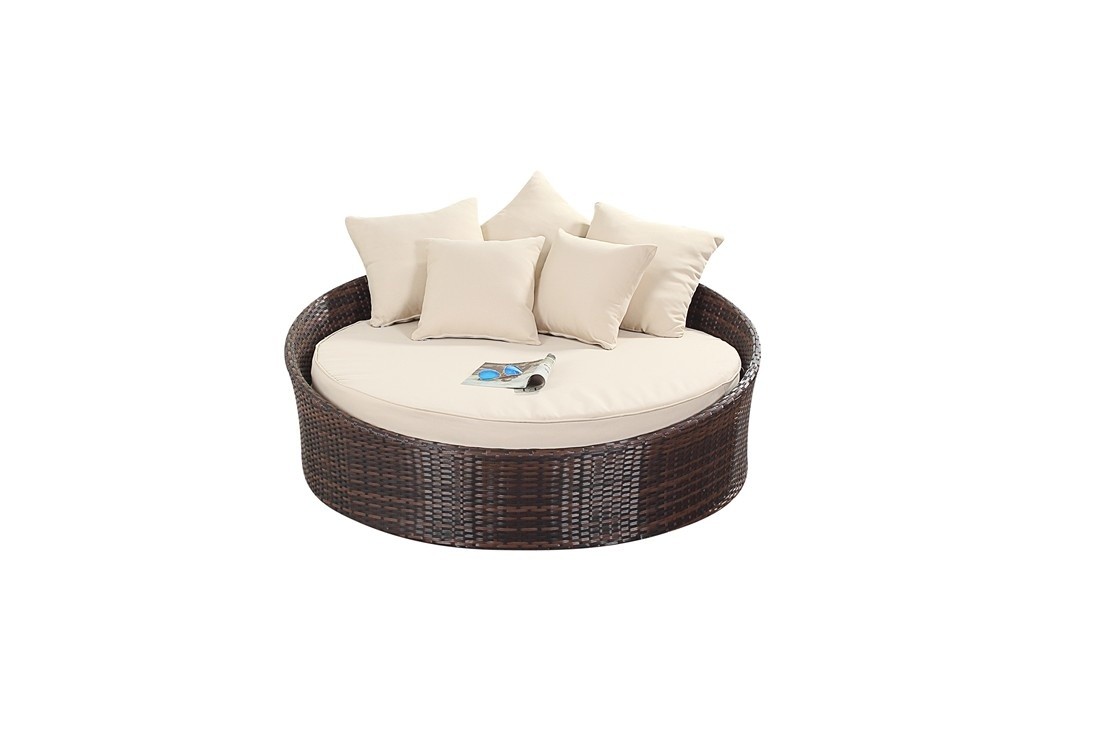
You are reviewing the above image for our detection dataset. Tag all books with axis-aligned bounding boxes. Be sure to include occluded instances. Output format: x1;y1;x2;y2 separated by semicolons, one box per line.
461;353;556;392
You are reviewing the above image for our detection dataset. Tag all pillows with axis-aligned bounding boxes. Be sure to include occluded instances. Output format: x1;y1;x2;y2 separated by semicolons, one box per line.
344;169;726;350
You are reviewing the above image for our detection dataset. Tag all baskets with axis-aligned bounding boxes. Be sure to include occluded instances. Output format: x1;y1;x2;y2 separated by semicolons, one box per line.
284;273;760;549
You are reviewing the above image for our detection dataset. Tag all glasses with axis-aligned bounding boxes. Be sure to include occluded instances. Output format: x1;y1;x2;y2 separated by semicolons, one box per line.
477;363;524;380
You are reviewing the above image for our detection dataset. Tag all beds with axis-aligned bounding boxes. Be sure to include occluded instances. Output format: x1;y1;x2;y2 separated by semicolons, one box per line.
283;274;762;548
